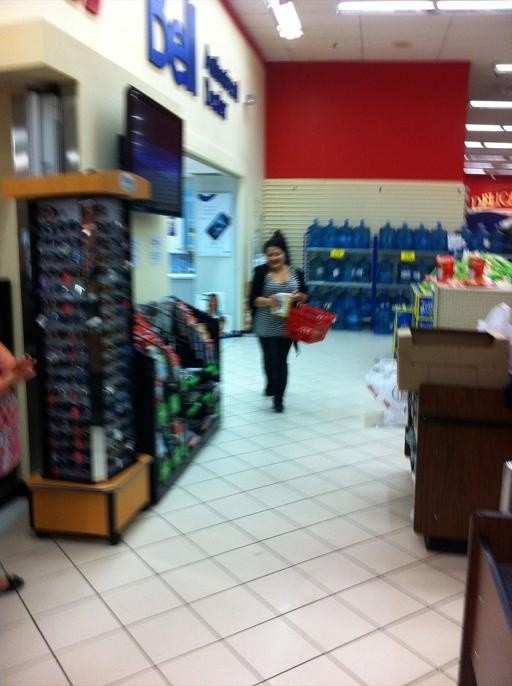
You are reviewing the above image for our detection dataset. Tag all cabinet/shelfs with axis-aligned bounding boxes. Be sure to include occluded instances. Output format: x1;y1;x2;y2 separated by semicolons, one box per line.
132;295;219;505
404;383;512;551
302;231;512;330
0;171;154;544
457;511;512;686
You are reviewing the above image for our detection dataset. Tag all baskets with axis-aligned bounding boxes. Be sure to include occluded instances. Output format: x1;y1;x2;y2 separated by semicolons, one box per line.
285;303;337;343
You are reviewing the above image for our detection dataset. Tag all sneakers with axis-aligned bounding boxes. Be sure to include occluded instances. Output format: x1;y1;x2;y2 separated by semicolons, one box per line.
265;379;284;413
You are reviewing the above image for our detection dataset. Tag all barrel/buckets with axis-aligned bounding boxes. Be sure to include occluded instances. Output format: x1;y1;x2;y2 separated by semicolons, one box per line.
304;216;506;336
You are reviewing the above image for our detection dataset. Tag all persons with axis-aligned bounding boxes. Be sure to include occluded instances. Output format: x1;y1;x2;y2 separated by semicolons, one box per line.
215;316;226;339
206;293;219;317
1;343;39;594
249;238;310;412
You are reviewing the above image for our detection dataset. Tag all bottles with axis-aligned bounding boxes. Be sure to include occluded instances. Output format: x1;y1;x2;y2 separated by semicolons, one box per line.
305;215;447;334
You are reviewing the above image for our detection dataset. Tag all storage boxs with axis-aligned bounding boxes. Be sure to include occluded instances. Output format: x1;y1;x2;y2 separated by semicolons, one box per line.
395;327;509;389
390;281;434;360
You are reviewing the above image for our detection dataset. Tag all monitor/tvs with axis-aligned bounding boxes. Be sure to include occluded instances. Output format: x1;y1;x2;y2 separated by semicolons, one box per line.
115;82;185;219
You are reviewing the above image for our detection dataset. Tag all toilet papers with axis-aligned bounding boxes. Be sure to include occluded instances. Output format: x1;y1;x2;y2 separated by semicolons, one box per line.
86;422;109;483
